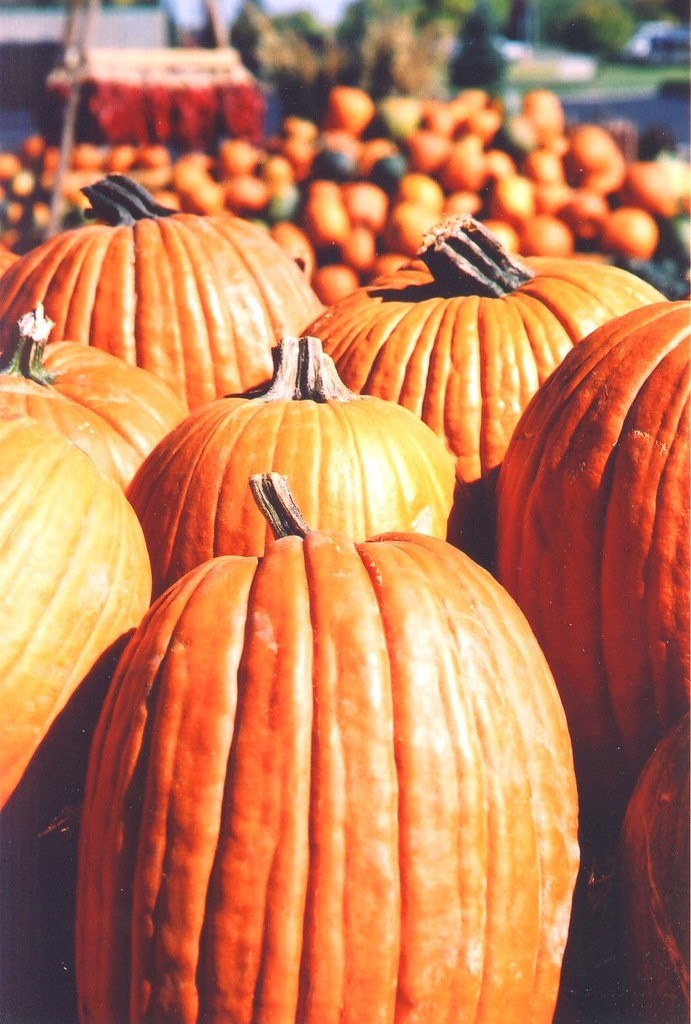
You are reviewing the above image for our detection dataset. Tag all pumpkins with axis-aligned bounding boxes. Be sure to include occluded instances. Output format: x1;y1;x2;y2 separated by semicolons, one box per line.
0;87;691;1024
